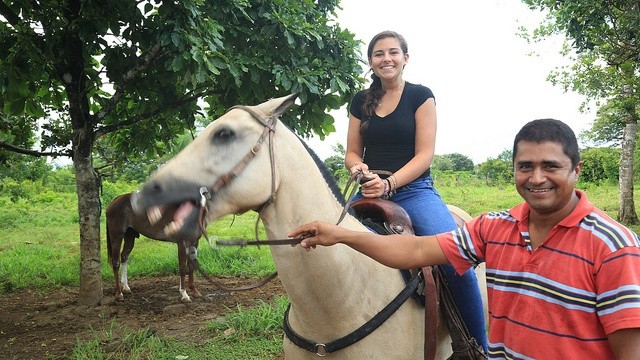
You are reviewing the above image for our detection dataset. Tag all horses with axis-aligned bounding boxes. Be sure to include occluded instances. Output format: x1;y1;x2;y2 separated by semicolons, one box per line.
106;193;204;304
127;92;488;360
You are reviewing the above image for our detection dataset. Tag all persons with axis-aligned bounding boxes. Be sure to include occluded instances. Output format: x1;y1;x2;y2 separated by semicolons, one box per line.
286;118;640;360
344;29;489;359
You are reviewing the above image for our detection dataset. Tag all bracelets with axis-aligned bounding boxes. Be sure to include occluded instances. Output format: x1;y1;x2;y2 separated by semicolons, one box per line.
384;175;397;199
